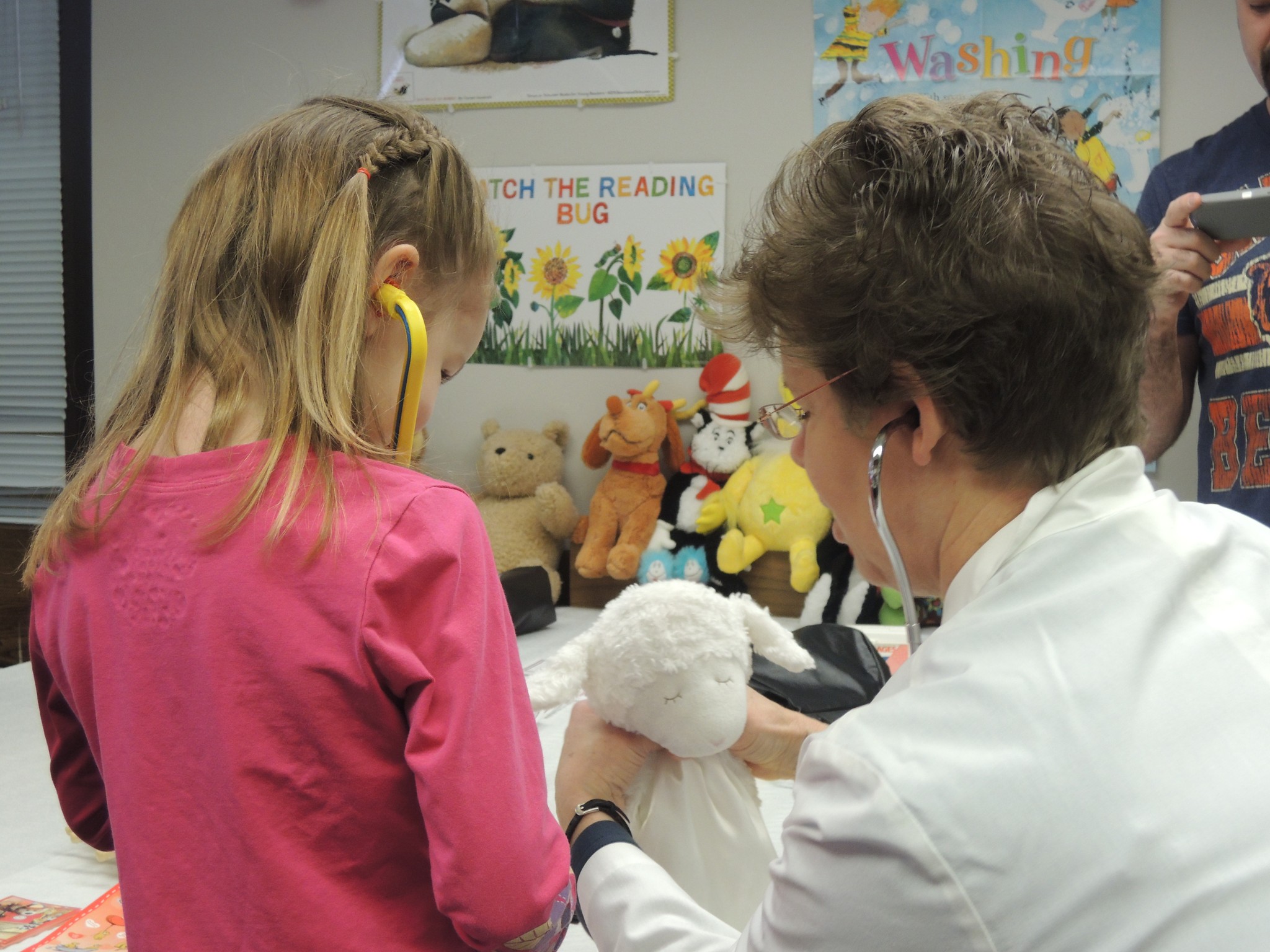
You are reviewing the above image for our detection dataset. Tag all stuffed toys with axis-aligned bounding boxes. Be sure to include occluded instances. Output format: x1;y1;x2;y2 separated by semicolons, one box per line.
526;579;816;932
468;353;941;627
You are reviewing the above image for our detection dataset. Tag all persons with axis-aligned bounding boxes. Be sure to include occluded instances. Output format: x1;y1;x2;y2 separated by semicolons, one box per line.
17;100;573;952
1128;0;1270;527
558;91;1270;952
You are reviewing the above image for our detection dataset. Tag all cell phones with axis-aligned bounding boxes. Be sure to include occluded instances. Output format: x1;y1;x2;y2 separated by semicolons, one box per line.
1188;187;1270;238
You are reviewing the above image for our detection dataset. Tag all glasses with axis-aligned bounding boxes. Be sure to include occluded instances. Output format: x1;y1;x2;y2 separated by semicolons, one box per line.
758;354;889;439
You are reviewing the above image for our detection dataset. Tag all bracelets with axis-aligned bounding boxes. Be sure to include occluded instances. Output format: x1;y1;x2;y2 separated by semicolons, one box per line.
565;799;633;844
